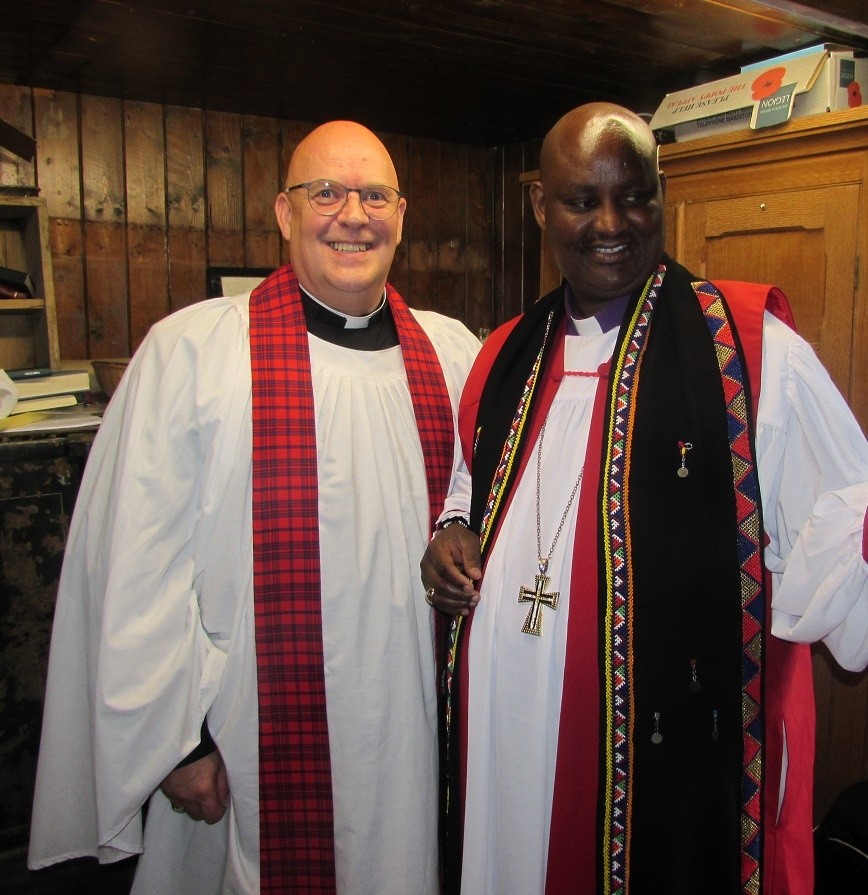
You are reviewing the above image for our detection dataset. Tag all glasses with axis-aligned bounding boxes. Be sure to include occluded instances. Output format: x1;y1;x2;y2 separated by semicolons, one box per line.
283;179;406;220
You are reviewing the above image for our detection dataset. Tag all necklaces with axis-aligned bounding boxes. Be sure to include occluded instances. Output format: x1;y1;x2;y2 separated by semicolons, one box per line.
515;423;591;639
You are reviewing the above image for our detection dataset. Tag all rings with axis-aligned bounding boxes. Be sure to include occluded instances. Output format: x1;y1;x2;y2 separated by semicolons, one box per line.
171;801;184;813
426;588;435;607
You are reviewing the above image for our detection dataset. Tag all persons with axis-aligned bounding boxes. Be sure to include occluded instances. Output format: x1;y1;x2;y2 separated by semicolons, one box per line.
29;120;492;895
421;103;868;895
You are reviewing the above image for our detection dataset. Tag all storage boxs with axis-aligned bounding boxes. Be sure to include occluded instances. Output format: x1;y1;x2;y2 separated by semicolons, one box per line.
649;43;868;145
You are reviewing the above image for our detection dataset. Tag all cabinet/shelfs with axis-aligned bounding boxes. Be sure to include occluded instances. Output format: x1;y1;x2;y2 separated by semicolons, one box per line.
515;97;867;819
0;197;113;895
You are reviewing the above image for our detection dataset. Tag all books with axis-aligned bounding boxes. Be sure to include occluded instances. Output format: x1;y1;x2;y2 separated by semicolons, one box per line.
4;366;89;416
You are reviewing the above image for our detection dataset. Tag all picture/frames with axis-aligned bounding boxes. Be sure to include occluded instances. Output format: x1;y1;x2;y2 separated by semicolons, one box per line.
208;267;278;300
